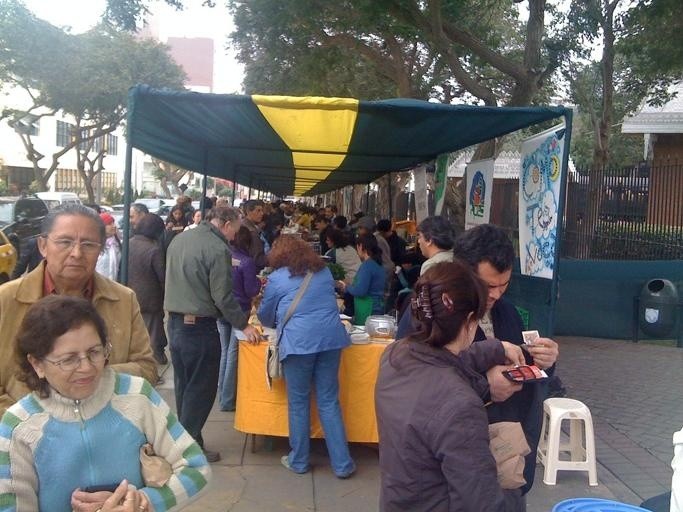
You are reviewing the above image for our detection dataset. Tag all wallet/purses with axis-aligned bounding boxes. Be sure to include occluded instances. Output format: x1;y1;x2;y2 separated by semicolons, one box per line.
502;365;550;384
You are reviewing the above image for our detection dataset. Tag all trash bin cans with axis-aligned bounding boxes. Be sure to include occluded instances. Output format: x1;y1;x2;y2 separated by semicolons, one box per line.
633;278;683;348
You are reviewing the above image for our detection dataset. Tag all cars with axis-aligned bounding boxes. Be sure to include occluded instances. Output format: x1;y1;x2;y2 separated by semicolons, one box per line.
0;190;270;284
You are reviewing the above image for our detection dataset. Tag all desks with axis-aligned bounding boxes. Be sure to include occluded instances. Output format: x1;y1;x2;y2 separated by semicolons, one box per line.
235;265;400;454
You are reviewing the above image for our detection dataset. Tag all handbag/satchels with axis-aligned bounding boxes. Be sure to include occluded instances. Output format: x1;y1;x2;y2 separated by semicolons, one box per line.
264;342;283;391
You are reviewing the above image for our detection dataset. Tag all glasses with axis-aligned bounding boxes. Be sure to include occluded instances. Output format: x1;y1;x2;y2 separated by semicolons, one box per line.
33;335;112;371
45;234;103;254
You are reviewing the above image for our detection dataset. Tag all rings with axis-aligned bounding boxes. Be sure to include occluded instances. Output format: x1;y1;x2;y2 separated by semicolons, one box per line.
76;504;83;510
137;504;145;510
124;498;135;502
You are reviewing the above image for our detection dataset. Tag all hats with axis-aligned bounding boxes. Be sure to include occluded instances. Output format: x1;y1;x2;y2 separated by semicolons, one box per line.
98;213;114;226
351;216;374;230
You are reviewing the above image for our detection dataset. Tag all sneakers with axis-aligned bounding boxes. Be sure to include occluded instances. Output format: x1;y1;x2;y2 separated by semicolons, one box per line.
204;450;218;462
280;455;290;469
153;351;167;365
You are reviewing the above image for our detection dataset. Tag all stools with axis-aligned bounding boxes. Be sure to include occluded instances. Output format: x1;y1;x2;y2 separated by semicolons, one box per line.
534;395;599;489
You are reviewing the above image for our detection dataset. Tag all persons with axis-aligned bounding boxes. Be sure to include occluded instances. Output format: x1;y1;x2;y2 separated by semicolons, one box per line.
256;233;357;479
162;205;264;463
8;195;458;411
373;261;526;512
395;223;558;512
0;294;212;512
0;203;159;424
